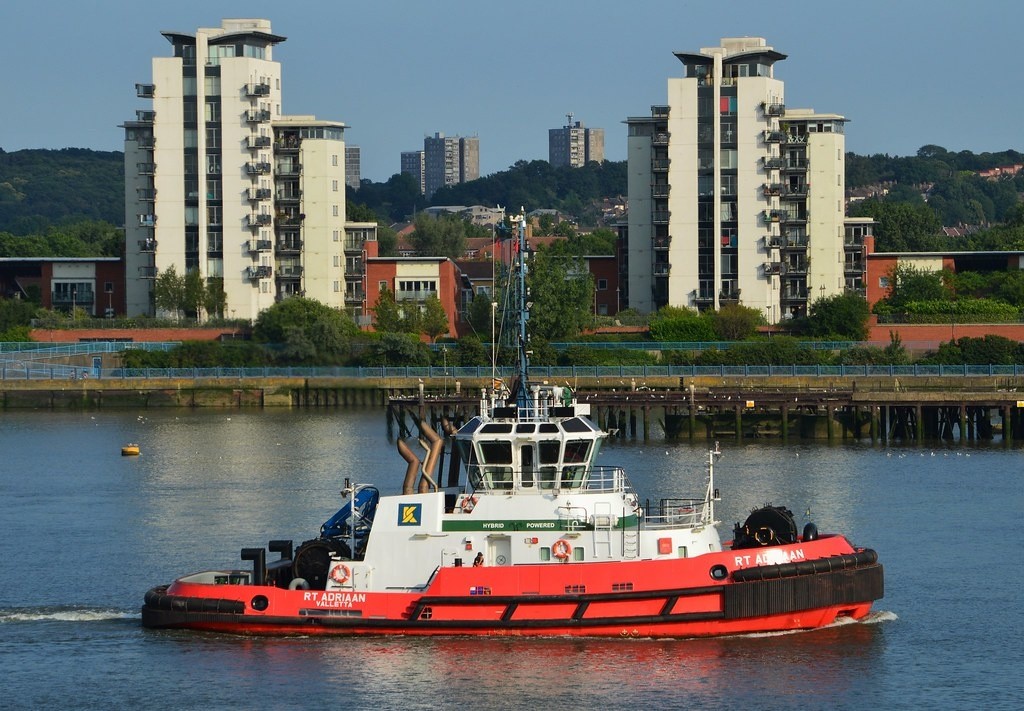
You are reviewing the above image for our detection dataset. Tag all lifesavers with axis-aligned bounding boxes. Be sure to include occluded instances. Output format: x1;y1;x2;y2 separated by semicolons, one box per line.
461;497;478;511
553;539;572;559
330;564;352;582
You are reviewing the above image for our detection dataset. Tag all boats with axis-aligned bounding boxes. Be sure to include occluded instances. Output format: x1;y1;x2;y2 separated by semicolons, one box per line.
138;205;886;640
120;442;142;458
